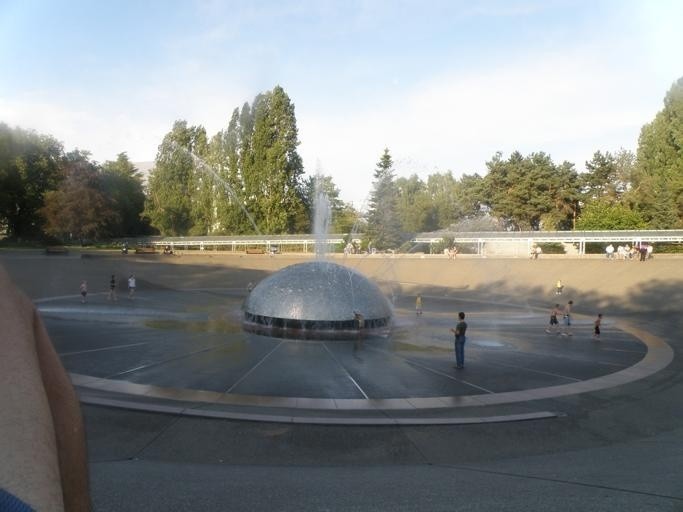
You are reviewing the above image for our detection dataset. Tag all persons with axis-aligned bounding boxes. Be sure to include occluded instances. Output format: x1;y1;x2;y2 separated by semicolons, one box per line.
121;242;128;254
416;292;422;314
343;239;375;256
544;303;563;335
531;243;543;259
450;312;467;370
163;246;174;255
1;264;92;511
108;275;120;300
593;313;603;340
82;280;88;305
555;279;564;295
603;243;654;260
127;276;137;296
246;281;255;292
444;246;458;261
564;300;573;335
